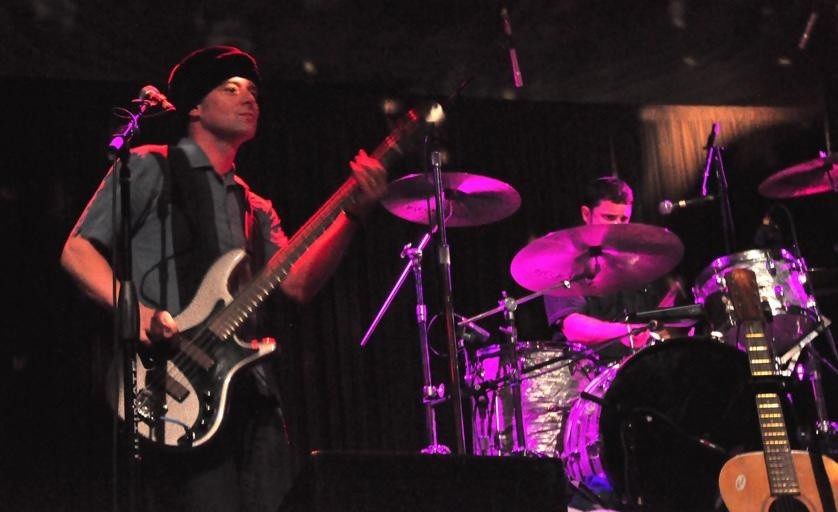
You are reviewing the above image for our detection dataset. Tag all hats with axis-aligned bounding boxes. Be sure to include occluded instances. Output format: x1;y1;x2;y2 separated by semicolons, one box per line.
167;45;262;115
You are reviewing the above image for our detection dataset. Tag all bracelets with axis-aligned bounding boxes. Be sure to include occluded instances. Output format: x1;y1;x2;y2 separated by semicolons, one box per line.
339;207;358;224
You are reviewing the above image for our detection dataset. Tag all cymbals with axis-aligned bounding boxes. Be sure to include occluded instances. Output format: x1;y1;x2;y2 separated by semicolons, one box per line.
759;157;838;199
380;172;521;229
511;222;684;296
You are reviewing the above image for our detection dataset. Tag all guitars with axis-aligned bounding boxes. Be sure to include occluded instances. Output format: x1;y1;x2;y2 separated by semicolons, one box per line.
105;100;445;448
717;266;838;512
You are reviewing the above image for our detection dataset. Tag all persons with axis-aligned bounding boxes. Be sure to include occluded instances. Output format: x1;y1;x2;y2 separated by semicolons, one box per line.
537;173;694;370
58;43;393;510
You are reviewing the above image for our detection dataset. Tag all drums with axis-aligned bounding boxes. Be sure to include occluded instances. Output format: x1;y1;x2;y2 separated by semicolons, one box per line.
472;341;604;459
693;246;820;353
557;337;801;511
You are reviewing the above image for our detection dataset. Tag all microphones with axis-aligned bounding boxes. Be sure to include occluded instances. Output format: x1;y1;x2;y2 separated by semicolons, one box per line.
139;84;177;115
658;192;714;216
650;320;666;331
